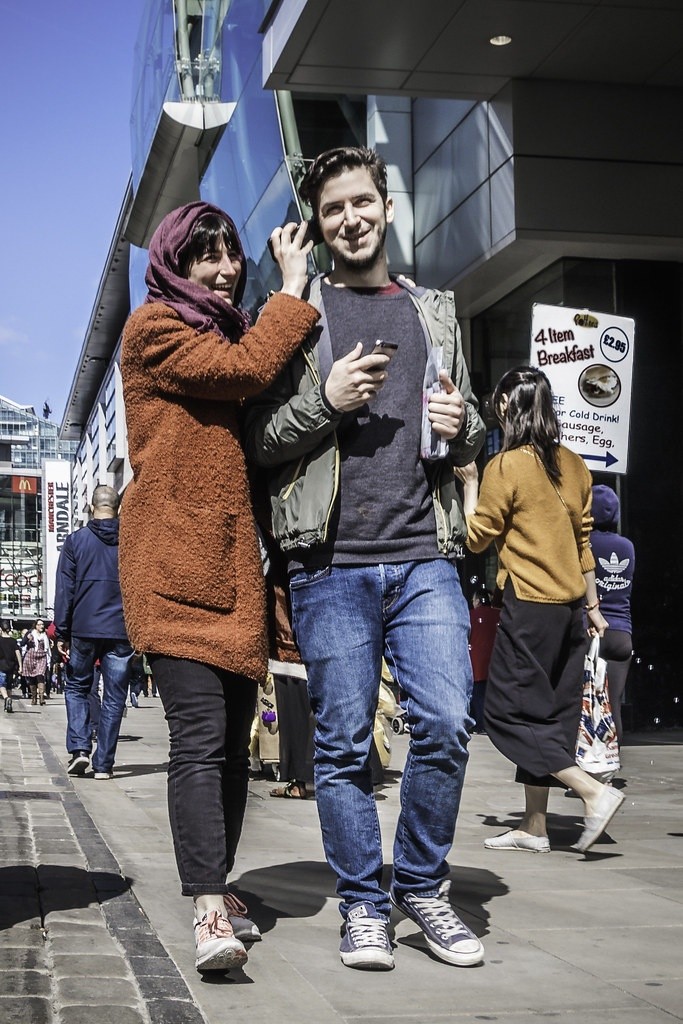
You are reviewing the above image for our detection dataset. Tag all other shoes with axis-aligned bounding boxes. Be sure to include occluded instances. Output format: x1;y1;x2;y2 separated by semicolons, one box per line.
269;780;308;800
4;697;13;712
131;692;139;707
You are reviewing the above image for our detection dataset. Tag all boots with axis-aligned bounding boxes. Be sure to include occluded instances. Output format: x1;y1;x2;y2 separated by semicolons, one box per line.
31;694;38;705
40;694;47;706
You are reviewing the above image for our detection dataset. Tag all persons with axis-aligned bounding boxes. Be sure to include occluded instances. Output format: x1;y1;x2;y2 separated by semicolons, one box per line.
52;485;136;779
561;483;634;797
116;202;317;973
454;366;626;852
258;146;487;970
1;563;385;800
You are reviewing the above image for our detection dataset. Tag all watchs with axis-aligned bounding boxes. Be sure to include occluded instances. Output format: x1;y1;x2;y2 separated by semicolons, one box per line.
583;600;600;611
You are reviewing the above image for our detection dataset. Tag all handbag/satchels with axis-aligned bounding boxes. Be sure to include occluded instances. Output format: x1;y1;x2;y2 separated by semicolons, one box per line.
574;631;623;777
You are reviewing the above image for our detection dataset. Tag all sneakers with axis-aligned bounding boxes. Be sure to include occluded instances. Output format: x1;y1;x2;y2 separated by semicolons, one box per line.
192;893;263;971
482;829;550;854
67;751;89;775
576;785;627;854
388;880;486;968
339;901;396;970
93;768;113;779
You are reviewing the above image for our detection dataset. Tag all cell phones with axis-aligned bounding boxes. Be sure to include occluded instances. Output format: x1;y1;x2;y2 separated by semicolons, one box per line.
267;219;325;263
363;340;398;374
